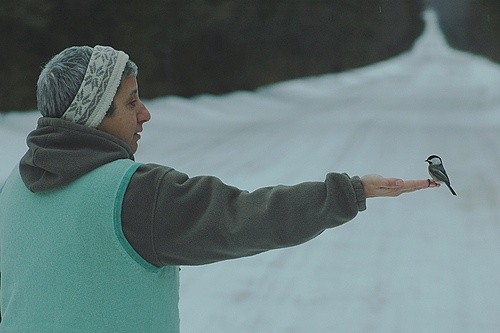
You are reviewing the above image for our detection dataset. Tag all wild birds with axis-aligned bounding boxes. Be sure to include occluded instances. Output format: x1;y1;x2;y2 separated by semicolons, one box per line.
424;154;457;196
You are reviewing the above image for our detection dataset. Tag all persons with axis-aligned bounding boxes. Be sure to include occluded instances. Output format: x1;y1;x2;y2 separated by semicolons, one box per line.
0;42;442;332
